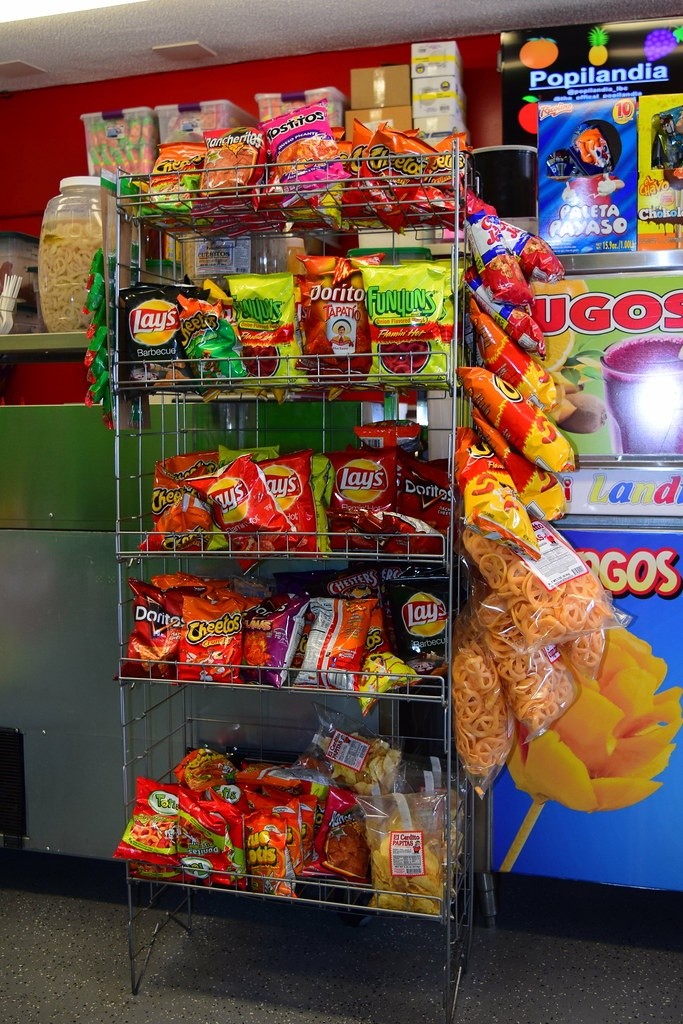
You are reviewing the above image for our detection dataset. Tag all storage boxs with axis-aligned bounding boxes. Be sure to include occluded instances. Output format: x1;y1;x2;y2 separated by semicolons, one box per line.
344;105;412;141
637;93;683;251
347;246;433;266
411;77;468;124
536;96;638;255
411;41;464;85
255;86;349;126
413;115;472;146
154;99;258;143
80;107;160;174
350;62;411;110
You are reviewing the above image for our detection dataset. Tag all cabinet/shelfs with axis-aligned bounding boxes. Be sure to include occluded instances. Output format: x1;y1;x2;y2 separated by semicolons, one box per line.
110;137;474;1024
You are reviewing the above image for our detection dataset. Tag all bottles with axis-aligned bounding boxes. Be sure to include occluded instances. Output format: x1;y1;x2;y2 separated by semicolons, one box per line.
36;175;103;330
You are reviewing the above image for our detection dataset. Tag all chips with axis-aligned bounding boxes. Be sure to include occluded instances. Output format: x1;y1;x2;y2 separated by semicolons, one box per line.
317;732;402;795
366;789;466;914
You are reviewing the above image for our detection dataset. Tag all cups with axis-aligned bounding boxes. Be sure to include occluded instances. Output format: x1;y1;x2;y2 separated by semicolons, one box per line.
472;144;537;219
601;334;682;458
